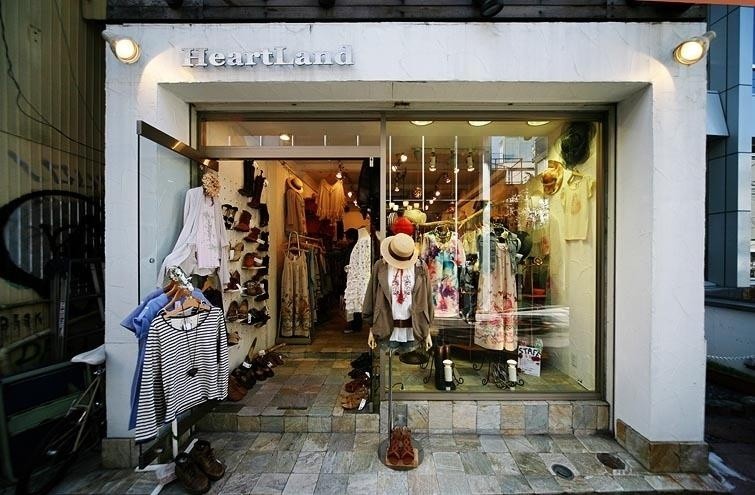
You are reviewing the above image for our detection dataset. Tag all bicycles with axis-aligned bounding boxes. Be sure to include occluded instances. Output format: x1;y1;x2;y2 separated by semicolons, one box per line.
43;332;106;474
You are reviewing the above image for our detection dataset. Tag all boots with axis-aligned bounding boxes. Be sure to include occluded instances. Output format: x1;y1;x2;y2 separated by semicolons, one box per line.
435;341;456;391
221;159;269;251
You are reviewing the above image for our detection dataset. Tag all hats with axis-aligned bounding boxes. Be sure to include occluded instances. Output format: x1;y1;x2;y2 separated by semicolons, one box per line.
379;233;418;269
286;177;304;194
391;217;415;237
541;160;564;196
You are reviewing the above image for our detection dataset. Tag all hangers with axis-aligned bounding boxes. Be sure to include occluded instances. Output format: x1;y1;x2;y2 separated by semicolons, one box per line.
566;167;583;184
430;213;514;243
279;239;325;255
156;266;211;322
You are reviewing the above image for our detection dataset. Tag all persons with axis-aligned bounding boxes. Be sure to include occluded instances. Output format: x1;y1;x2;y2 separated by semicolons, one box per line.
344;225;376;316
421;225;466;319
475;226;522;353
368;255;435;353
339;228;362;335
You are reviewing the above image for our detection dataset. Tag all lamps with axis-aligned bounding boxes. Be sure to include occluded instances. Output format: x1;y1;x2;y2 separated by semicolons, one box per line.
335;153;460;213
100;28;143;68
528;121;550;126
428;149;477;173
468;121;492;127
673;30;717;67
410;120;433;126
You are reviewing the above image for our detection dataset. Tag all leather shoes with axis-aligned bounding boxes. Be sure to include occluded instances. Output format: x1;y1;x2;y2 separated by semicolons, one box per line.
225;242;270;345
341;350;373;410
226;351;284;401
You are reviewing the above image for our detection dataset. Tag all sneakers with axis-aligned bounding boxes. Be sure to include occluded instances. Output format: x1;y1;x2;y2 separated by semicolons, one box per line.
175;440;227;495
344;326;359;334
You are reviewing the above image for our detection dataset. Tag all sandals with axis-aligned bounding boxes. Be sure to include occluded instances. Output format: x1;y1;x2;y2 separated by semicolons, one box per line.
388;423;415;466
